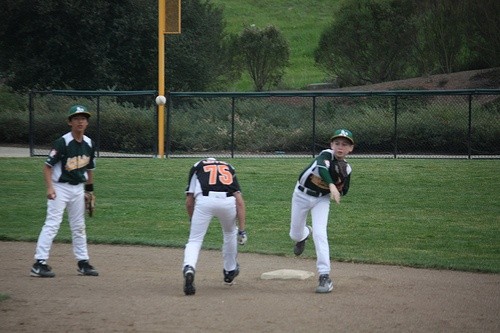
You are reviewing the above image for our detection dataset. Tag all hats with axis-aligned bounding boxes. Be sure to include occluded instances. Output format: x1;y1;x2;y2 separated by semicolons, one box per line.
67;105;91;119
331;129;354;144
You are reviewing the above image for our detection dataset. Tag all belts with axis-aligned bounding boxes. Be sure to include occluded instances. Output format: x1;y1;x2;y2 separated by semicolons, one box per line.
58;180;80;185
202;191;234;197
298;186;327;198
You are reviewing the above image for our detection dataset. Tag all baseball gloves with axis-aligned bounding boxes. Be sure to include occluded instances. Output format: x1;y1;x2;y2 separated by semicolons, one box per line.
84;190;95;217
329;160;352;185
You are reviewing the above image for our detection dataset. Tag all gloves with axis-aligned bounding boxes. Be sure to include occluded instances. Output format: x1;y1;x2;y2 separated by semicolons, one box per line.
239;231;247;245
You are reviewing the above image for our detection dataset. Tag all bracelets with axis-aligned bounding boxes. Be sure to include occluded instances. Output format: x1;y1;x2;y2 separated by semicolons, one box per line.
238;230;246;235
84;184;93;191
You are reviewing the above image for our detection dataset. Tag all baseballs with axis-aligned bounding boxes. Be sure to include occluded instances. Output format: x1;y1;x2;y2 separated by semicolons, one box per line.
155;95;167;105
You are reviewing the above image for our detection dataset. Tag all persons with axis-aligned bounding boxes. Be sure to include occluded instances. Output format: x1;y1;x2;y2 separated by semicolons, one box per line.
181;157;247;296
288;129;355;294
30;105;102;278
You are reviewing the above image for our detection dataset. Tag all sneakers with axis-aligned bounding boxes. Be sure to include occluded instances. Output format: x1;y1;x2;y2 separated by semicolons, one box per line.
294;225;312;255
316;274;333;292
30;260;55;278
183;266;196;295
77;260;98;276
223;263;240;284
